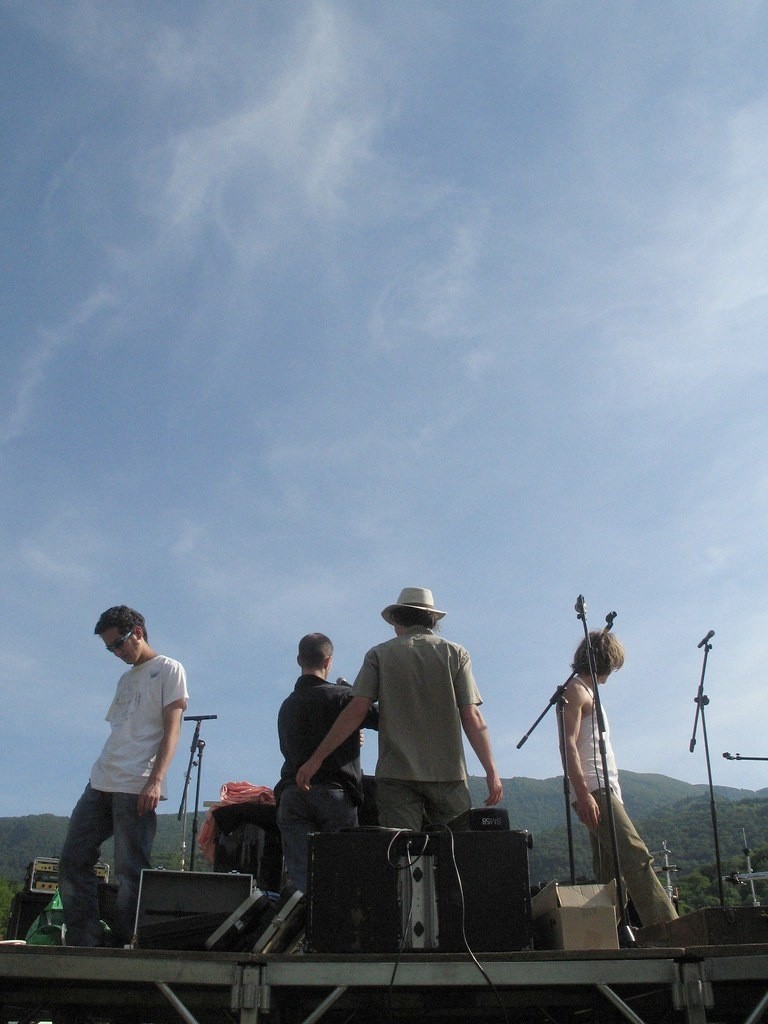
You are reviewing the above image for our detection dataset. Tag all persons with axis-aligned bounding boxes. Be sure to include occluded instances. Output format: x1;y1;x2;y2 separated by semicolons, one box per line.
295;586;504;831
274;632;379;893
58;605;191;946
555;632;680;926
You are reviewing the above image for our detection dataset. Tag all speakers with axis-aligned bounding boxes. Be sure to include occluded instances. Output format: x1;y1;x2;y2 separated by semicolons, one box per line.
7;892;56;941
307;829;537;953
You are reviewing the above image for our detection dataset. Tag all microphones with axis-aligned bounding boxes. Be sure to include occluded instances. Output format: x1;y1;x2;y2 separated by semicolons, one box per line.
698;630;716;649
184;715;217;721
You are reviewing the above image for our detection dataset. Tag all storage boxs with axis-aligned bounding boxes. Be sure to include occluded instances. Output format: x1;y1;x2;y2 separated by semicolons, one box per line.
131;866;253;951
532;878;620;950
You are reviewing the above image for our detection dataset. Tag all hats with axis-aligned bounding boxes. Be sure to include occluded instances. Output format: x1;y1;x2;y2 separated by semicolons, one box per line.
381;587;447;626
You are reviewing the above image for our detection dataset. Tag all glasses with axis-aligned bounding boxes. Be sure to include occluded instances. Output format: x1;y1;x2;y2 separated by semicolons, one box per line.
107;630;134;653
